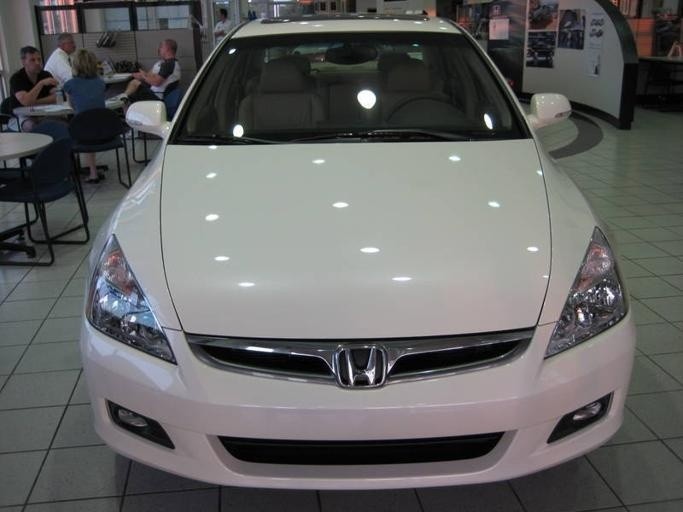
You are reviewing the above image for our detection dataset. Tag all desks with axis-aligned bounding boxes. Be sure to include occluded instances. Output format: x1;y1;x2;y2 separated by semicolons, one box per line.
638;57;683;113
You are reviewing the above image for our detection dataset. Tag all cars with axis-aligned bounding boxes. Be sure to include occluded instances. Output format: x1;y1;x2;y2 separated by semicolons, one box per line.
527;41;556;57
80;11;636;495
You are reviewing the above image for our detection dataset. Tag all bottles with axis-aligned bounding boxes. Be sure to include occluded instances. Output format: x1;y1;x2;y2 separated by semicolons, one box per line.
54;86;64;106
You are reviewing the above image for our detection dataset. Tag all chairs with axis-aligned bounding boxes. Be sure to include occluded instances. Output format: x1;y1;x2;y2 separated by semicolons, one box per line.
0;61;182;267
239;52;464;132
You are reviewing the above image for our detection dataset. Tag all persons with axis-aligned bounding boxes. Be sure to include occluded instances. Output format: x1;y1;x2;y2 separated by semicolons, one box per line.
213;8;232;49
103;39;181;111
61;48;107;184
6;45;59;161
42;32;77;92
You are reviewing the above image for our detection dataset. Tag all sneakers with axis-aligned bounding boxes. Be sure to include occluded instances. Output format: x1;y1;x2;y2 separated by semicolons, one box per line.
80;166;99;183
105;94;129;110
